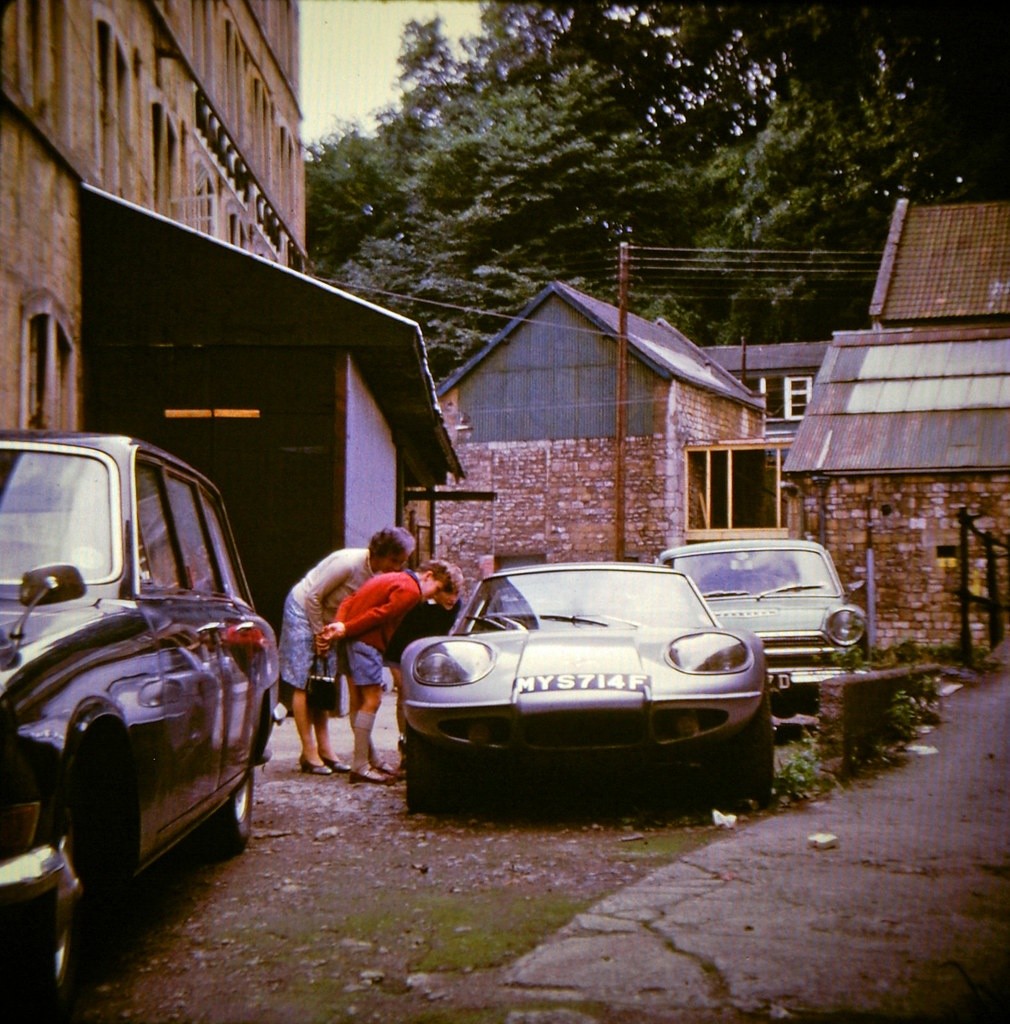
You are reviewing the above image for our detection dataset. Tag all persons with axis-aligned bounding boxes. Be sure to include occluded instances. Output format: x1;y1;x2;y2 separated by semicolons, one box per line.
320;557;471;785
278;526;417;776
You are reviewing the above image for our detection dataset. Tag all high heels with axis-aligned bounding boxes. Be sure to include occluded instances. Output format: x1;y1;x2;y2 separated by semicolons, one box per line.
299;754;333;775
322;757;352;773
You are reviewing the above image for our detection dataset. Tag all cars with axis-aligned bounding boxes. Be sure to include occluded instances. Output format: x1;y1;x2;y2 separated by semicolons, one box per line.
659;540;868;718
0;429;284;1024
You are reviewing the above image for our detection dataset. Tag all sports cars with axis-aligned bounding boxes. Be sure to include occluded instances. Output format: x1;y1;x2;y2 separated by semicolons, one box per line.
402;561;776;814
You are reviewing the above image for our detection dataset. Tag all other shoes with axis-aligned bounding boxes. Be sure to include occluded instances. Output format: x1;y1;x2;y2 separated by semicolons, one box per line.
350;764;396;785
371;760;408;780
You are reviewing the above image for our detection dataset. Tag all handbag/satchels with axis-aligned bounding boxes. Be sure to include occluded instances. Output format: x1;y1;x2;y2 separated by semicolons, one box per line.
306;654;338;711
